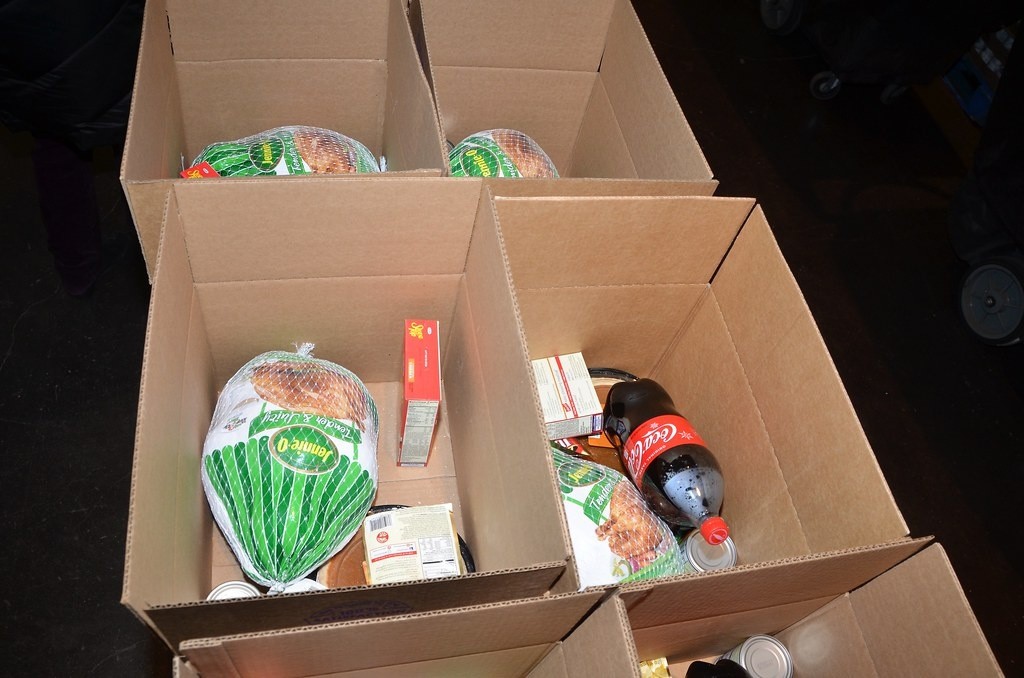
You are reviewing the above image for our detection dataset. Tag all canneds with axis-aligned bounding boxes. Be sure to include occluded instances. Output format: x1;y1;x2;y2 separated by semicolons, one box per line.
679;528;737;574
205;580;260;600
714;633;794;678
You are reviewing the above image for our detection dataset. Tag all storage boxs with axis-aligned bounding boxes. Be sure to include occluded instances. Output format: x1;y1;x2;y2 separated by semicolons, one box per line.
120;2;1004;678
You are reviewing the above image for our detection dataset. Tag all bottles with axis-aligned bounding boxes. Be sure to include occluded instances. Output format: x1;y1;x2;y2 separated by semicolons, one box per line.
602;378;728;545
